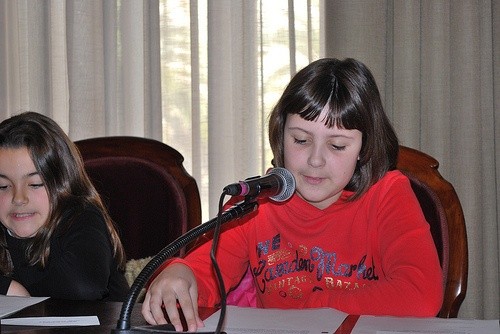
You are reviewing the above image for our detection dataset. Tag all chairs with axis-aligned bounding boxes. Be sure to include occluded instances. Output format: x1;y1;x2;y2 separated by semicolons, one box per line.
265;145;469;318
70;135;202;254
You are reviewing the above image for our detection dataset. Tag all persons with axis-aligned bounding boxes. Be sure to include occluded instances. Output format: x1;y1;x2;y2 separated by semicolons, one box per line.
141;57;444;334
0;111;131;303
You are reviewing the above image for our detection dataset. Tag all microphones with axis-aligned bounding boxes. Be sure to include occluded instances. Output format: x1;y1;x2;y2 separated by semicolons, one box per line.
223;167;297;202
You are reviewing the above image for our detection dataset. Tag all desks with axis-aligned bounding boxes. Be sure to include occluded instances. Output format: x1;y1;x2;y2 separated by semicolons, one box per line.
0;296;496;334
